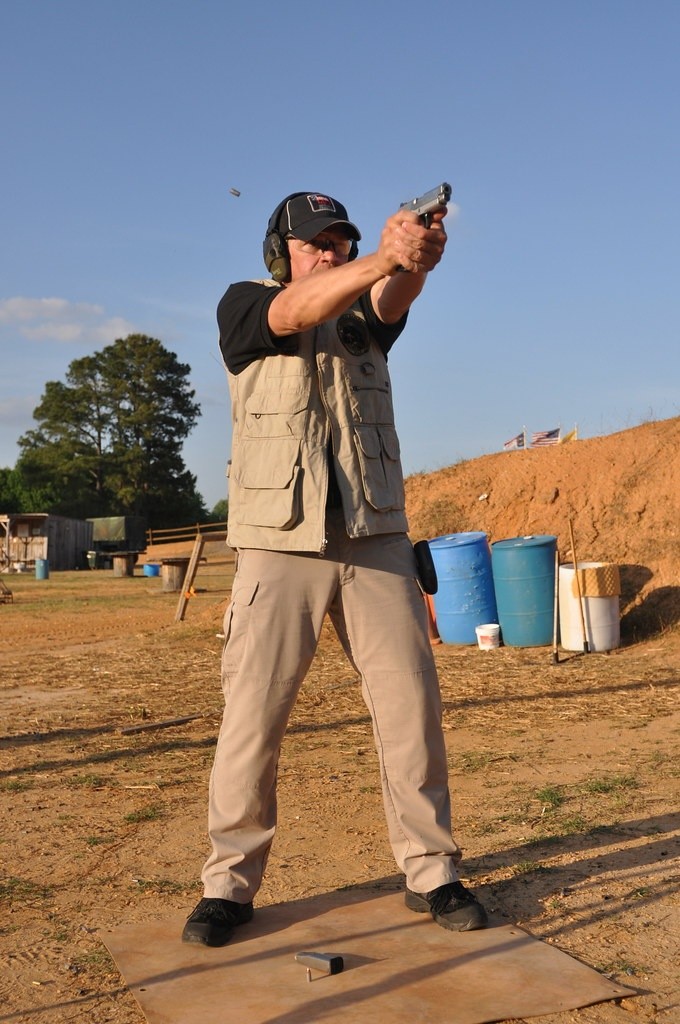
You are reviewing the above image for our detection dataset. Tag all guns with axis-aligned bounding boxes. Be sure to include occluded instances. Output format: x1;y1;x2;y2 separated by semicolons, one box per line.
395;181;454;273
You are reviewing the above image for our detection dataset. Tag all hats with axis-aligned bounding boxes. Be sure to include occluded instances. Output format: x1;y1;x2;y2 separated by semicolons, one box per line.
265;194;361;250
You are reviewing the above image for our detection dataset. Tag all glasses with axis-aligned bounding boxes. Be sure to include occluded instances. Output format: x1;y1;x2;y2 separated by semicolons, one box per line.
285;233;352;257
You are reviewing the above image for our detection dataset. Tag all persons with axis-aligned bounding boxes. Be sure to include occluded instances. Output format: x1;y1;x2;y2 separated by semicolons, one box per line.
180;193;489;948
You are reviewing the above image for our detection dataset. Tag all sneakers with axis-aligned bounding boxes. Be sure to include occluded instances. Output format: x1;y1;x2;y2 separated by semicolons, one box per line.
406;875;488;932
181;897;253;946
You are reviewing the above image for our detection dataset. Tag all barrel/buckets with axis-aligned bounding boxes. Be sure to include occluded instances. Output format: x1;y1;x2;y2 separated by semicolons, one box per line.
477;623;499;649
428;531;501;645
559;562;619;651
36;560;49;579
490;535;560;647
144;565;159;576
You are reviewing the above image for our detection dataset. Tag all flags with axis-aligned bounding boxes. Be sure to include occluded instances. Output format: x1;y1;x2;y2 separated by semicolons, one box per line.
530;428;560;448
561;428;576;444
504;432;524;448
